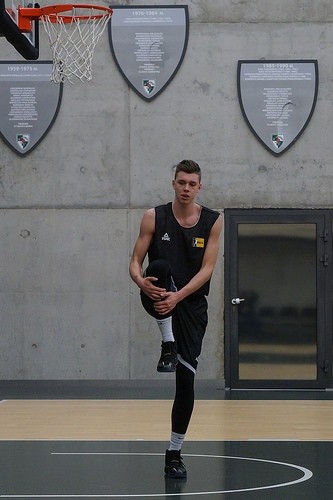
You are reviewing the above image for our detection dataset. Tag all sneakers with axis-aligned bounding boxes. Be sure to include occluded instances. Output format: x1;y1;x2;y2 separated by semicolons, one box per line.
165;449;187;477
157;339;178;372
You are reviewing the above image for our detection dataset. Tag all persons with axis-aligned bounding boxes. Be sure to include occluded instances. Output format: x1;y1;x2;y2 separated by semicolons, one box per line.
128;159;224;480
